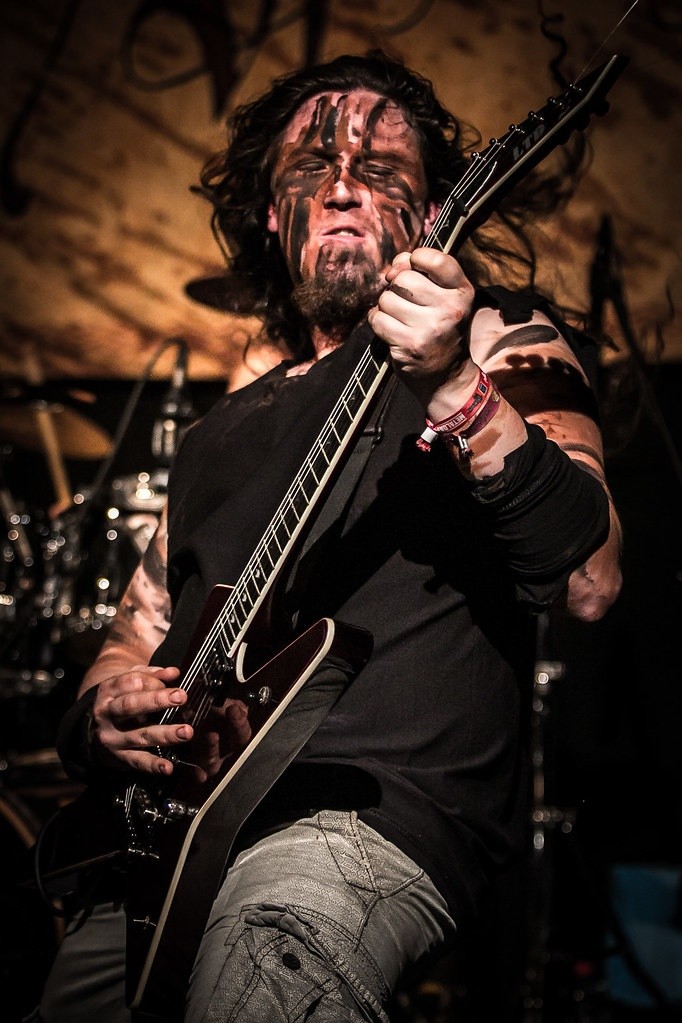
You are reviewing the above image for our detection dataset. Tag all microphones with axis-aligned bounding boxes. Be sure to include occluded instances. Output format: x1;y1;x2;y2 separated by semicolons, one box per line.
153;347;192;462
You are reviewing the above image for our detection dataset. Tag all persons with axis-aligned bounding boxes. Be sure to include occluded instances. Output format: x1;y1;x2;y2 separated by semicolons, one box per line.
43;46;623;1023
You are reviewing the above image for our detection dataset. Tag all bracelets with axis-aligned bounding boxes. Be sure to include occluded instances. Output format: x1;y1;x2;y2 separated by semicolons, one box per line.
441;379;501;462
416;368;490;453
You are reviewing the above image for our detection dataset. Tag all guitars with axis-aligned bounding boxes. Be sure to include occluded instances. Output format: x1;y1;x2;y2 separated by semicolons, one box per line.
98;52;621;1011
